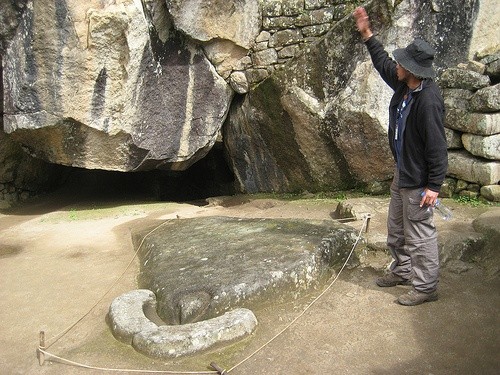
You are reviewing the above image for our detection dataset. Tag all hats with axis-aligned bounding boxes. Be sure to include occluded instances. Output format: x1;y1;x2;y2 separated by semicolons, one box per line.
393;39;435;78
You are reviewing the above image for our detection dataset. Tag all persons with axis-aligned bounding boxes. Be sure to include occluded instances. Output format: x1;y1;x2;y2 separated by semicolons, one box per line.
353;6;449;305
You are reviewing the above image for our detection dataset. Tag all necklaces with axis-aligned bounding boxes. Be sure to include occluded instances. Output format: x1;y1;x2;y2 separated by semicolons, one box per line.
394;86;421;141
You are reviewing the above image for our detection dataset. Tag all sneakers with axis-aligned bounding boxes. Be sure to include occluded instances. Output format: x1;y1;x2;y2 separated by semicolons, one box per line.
399;288;440;306
376;272;411;287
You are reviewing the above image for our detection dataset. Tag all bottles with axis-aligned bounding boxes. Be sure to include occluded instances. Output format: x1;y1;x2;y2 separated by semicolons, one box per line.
420;191;453;220
